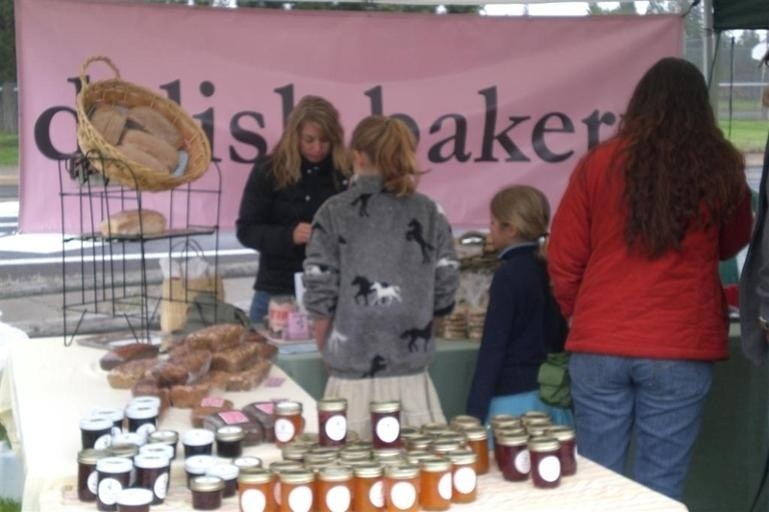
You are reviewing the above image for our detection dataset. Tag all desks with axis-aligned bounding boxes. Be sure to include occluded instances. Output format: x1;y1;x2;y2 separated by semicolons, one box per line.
429;338;479;422
447;455;687;510
17;328;320;511
256;323;329;402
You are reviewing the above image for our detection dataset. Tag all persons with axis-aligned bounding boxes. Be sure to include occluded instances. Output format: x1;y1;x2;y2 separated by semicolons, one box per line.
737;32;768;511
234;93;353;323
467;185;574;453
553;58;752;505
299;115;460;434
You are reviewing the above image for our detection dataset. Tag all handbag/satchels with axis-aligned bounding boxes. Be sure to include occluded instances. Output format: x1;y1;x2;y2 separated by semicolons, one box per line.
537;351;572;408
160;238;226;334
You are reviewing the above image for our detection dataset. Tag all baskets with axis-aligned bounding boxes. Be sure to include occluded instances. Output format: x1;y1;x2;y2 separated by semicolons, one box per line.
76;55;211;194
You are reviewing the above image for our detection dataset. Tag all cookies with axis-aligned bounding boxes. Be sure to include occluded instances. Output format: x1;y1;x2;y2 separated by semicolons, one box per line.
442;306;488;341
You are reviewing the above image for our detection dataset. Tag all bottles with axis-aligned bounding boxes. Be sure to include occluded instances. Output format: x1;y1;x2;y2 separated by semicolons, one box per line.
238;397;578;512
218;423;244;458
182;428;214;457
184;453;263;511
73;397;178;512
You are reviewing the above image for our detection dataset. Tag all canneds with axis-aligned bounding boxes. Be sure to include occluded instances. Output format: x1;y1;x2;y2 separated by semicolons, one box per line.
267;294;299;339
76;395;578;512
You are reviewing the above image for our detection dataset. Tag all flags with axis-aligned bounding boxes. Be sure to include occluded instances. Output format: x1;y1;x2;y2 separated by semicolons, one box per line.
12;1;688;234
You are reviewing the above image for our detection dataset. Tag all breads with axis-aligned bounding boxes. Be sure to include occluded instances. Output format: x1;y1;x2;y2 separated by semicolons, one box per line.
90;104;185;176
100;207;167;238
99;323;278;447
